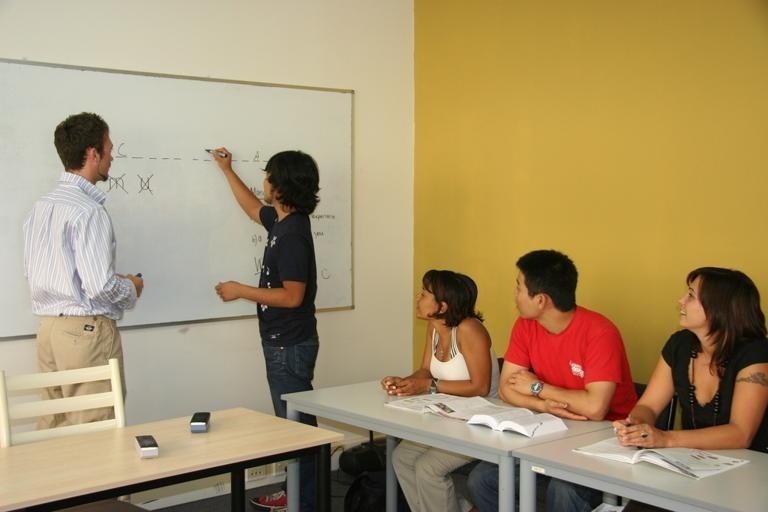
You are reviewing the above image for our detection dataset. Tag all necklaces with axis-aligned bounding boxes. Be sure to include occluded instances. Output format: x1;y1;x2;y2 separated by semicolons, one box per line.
687;337;722;429
437;326;453;360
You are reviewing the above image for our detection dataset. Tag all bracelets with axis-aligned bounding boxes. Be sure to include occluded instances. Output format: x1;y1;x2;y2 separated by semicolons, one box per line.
429;377;438;394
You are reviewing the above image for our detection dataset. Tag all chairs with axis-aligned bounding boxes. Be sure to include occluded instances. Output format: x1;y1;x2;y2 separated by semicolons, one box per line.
0;358;152;512
628;382;679;439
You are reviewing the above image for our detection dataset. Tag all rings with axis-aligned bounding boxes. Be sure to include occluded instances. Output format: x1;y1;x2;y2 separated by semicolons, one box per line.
641;430;648;439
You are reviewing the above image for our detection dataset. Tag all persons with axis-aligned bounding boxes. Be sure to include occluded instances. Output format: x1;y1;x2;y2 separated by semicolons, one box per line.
612;266;768;452
208;145;321;511
23;112;145;429
380;268;501;512
466;249;639;512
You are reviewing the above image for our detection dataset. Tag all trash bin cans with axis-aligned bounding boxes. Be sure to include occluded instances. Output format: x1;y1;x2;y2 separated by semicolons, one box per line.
337;437;409;512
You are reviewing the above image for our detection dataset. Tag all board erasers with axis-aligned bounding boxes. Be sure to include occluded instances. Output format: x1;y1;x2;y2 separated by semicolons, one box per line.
191;412;211;433
135;435;159;458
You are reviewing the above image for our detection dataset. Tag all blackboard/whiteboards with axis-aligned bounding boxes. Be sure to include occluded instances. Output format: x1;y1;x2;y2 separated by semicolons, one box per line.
0;59;353;342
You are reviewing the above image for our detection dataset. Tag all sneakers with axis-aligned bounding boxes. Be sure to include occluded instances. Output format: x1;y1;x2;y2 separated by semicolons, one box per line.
248;490;287;509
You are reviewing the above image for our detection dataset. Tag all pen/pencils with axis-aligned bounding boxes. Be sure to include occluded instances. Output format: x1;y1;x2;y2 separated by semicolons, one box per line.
136;273;142;277
205;149;227;158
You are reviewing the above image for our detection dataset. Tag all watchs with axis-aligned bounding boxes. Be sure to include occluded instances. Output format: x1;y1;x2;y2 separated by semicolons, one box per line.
529;379;545;397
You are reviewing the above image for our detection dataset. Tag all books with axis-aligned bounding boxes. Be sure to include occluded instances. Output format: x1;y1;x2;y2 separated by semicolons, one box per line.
571;435;753;482
383;392;496;421
467;408;568;438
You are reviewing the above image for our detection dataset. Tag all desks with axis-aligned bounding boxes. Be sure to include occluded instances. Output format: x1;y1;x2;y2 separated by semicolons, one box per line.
280;378;618;512
1;406;342;512
509;422;766;512
